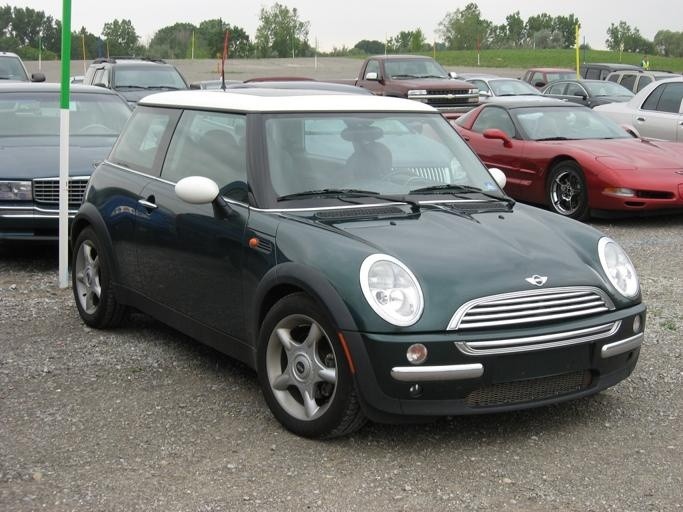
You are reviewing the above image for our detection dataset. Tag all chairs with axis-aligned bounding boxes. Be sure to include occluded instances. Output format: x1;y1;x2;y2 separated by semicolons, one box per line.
185;131;391;190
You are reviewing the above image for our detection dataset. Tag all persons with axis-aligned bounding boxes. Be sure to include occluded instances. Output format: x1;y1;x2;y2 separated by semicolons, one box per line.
640;55;651;70
